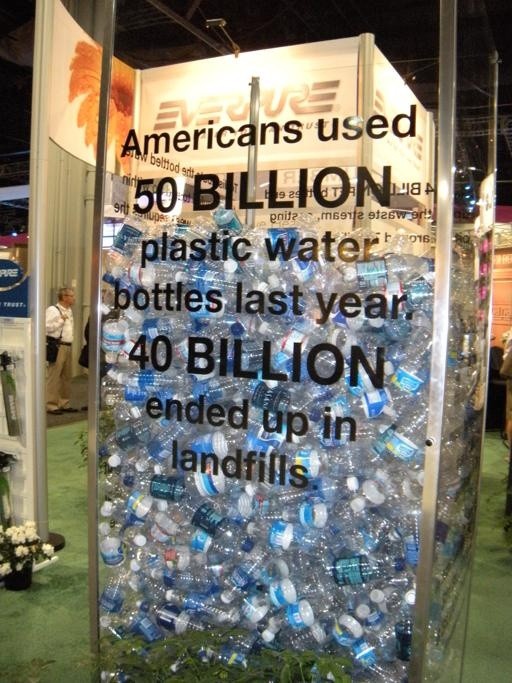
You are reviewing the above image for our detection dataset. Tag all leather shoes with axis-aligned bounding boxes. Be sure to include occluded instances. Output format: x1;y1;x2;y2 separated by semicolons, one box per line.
47;407;78;415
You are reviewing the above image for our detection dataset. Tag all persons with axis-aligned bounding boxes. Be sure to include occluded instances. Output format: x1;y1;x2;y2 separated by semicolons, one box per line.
497;330;511;516
77;286;120;412
43;286;76;415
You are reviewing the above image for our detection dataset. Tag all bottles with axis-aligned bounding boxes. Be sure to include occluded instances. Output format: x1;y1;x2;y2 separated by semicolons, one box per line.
100;204;491;681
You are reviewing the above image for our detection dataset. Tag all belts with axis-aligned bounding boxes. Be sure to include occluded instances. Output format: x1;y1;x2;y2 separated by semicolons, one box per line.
60;342;71;346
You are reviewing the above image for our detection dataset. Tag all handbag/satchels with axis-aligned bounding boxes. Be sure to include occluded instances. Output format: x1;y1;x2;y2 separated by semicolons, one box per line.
46;343;58;362
79;345;88;368
